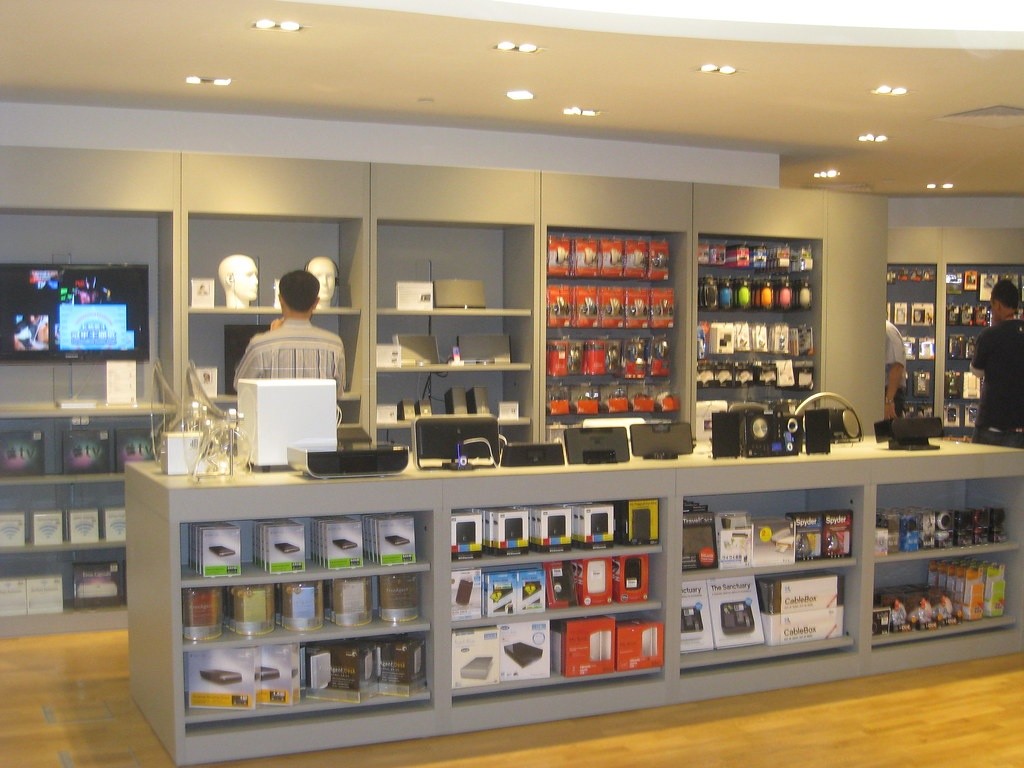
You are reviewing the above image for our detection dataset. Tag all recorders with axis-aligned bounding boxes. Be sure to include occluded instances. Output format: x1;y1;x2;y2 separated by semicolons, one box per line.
794;392;866;443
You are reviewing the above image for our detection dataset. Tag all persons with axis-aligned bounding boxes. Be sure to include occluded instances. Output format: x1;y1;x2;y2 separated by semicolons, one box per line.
218;254;258;308
197;285;209;296
885;319;906;420
203;372;210;384
969;279;1024;449
234;270;347;401
15;314;40;350
304;256;344;310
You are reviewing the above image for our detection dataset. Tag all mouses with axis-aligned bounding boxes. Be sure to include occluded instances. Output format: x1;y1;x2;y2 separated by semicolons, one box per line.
699;282;812;385
548;245;674;407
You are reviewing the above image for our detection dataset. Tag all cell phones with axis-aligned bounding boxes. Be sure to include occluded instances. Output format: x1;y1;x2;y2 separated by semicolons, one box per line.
276;543;300;553
210;546;235;557
385;535;410;545
333;539;357;550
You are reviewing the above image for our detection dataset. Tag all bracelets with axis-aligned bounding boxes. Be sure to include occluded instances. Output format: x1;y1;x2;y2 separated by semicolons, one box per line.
886;397;894;404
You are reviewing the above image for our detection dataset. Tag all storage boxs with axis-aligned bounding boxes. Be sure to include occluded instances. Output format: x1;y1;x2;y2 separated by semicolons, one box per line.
188;499;851;719
0;429;156;619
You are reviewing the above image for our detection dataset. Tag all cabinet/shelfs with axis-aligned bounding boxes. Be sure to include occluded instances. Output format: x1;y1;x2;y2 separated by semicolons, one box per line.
1;401;181;638
124;440;1024;768
181;151;371;448
372;161;542;451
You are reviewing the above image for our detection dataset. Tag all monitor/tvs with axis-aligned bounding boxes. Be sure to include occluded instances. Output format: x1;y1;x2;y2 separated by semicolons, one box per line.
0;262;150;365
